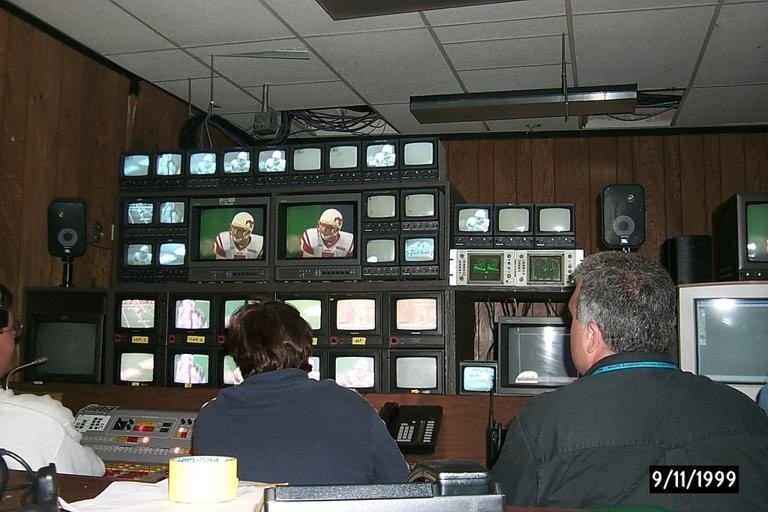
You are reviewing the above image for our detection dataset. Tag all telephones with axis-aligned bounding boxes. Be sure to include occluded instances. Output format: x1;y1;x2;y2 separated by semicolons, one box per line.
378;402;443;454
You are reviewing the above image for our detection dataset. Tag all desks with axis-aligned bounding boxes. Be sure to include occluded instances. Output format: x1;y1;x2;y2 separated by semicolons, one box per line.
0;470;118;512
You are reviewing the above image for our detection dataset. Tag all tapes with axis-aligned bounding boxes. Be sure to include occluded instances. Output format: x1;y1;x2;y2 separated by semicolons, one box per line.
168;457;237;502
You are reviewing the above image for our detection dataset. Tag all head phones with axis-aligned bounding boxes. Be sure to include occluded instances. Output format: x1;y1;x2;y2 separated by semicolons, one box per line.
0;448;58;512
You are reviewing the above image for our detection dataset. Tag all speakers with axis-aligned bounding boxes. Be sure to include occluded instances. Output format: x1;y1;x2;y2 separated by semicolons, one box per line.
47;196;87;258
19;286;110;385
601;183;646;248
661;235;713;285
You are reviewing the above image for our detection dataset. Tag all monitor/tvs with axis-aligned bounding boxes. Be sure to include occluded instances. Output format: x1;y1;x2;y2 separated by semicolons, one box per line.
498;316;583;396
454;203;493;249
676;281;768;403
536;204;576;248
22;312;105;385
712;193;768;280
450;249;515;286
493;203;534;248
109;135;450;394
459;361;498;394
515;250;584;287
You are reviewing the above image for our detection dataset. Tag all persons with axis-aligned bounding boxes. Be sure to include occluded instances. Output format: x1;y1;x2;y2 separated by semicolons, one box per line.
338;358;374;389
372;145;393;167
300;208;354;259
190;298;413;486
465;209;492;232
263;151;286;169
196;155;216;175
487;252;768;512
213;211;265;261
231;152;251;171
133;244;152;264
0;283;106;478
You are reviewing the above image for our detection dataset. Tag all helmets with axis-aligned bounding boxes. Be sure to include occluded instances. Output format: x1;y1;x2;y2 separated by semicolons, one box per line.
476;211;486;219
203;155;212;161
382;145;392;153
272;151;281;159
238;152;247;160
231;212;254;244
318;209;343;242
140;245;149;253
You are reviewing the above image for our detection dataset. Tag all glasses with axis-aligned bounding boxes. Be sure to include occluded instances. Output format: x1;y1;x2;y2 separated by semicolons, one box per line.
3;322;23;338
